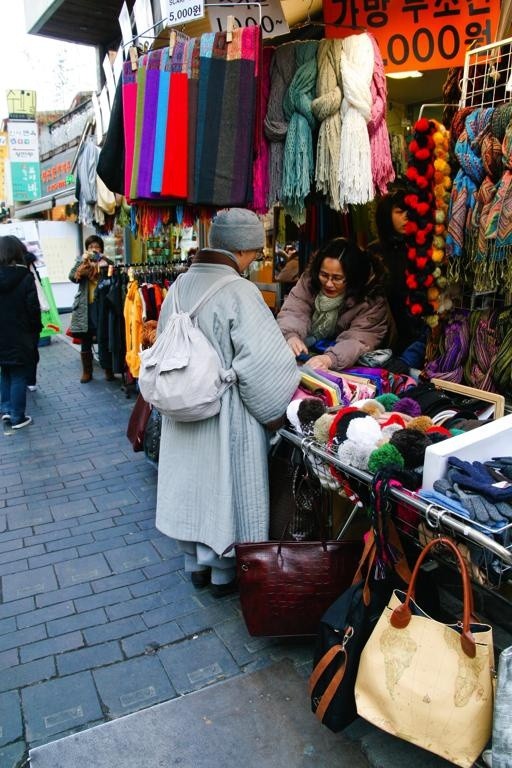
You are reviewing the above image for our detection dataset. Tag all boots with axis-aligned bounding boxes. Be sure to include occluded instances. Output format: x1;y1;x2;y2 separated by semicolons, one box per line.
104;367;116;382
78;350;94;385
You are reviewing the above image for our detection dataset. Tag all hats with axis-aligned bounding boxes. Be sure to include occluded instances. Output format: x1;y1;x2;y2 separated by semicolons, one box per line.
207;208;267;252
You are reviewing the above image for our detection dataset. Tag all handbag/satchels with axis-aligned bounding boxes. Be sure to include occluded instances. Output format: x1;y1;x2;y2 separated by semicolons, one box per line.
483;643;512;767
126;392;151;456
140;403;161;465
306;512;418;736
349;531;494;767
230;473;355;643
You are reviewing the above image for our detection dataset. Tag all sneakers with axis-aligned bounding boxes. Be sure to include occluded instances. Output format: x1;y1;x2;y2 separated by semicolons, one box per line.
1;412;32;435
210;581;239;598
190;567;211;590
26;385;37;391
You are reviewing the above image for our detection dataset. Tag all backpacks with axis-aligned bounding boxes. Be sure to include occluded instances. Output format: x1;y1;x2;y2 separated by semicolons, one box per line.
136;271;245;425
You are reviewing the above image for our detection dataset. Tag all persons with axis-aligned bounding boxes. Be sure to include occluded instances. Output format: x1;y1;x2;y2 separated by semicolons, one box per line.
277;237;398;370
366;187;409;255
69;235;114;383
0;235;44;430
138;207;301;599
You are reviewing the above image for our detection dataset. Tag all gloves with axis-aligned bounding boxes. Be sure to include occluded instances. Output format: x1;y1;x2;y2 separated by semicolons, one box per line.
431;453;512;526
